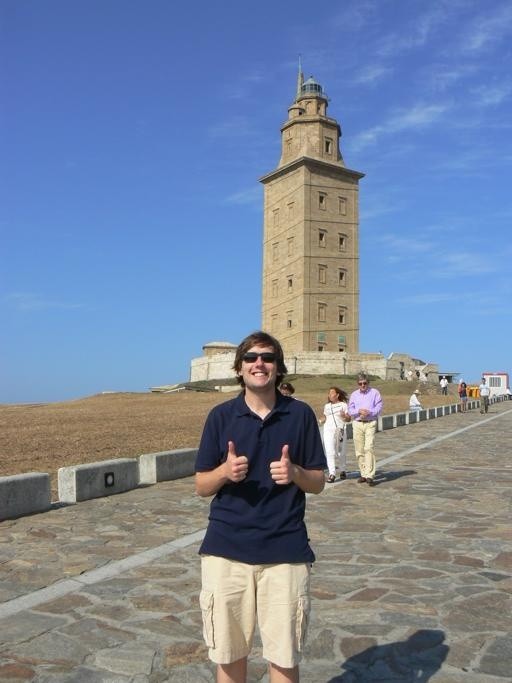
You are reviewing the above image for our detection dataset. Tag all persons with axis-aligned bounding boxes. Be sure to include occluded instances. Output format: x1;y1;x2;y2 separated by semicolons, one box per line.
320;387;351;483
507;387;512;400
440;376;448;395
410;390;422;410
195;333;328;683
458;383;467;413
479;378;490;414
280;383;295;397
348;374;383;483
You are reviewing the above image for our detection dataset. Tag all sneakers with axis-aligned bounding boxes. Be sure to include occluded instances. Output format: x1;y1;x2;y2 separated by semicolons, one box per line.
340;472;346;478
327;475;336;482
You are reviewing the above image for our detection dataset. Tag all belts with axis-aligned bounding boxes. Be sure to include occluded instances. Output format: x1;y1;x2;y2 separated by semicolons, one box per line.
359;419;372;421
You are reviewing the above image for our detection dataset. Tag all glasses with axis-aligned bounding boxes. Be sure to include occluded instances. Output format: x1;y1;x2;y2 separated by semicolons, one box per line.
241;353;276;363
358;382;367;385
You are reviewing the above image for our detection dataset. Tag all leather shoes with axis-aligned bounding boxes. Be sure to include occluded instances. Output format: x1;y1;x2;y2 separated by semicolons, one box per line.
367;478;374;485
358;477;366;482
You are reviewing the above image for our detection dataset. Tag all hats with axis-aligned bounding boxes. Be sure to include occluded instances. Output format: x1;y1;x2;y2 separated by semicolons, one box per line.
413;389;422;393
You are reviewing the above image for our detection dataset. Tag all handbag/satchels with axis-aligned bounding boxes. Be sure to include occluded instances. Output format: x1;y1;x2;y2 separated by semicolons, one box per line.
334;427;344;441
